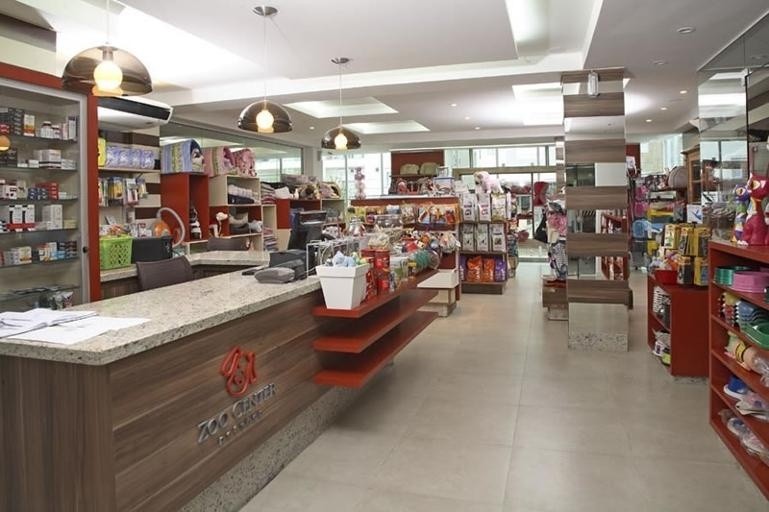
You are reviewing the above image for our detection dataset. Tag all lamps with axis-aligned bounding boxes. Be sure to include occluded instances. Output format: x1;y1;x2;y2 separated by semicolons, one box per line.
236;3;363;150
57;1;153;100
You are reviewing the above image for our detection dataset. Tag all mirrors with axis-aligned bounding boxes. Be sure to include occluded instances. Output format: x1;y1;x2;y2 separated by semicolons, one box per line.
560;80;629;282
693;70;749;218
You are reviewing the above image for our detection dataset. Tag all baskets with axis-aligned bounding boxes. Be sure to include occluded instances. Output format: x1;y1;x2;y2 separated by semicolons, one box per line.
98;233;133;270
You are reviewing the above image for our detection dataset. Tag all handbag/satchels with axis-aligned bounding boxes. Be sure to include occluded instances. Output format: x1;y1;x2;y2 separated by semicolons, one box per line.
420;162;440;176
667;166;687;187
400;164;419;174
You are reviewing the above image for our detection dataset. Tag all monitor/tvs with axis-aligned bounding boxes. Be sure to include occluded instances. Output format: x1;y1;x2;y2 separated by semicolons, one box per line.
287;211;328;250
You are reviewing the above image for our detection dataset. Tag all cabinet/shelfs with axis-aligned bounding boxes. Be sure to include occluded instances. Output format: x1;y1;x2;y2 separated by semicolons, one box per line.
706;172;769;497
2;71;173;310
458;187;518;295
311;196;462;387
632;174;710;379
389;151;445;195
162;171;348;265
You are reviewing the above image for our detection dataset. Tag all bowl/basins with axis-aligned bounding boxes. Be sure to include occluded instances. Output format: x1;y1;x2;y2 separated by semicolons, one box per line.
730;270;769;293
714;265;751;286
